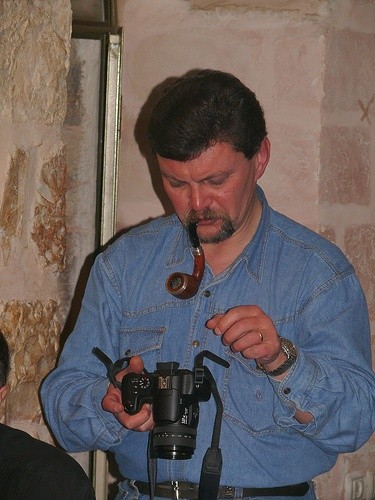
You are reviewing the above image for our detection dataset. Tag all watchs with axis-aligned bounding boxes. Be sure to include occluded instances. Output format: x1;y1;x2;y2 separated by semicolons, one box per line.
254;336;298;376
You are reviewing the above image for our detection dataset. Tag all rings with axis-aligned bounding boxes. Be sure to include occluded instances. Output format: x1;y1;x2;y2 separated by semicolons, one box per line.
255;330;265;342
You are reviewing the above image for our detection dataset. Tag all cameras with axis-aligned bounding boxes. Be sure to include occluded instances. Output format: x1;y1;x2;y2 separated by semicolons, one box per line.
121;361;211;460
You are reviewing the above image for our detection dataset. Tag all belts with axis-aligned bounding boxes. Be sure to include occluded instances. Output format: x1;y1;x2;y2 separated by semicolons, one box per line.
134;480;310;499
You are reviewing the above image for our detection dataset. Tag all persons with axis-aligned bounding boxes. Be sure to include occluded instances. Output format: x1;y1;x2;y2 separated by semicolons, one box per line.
36;67;375;499
1;326;94;500
